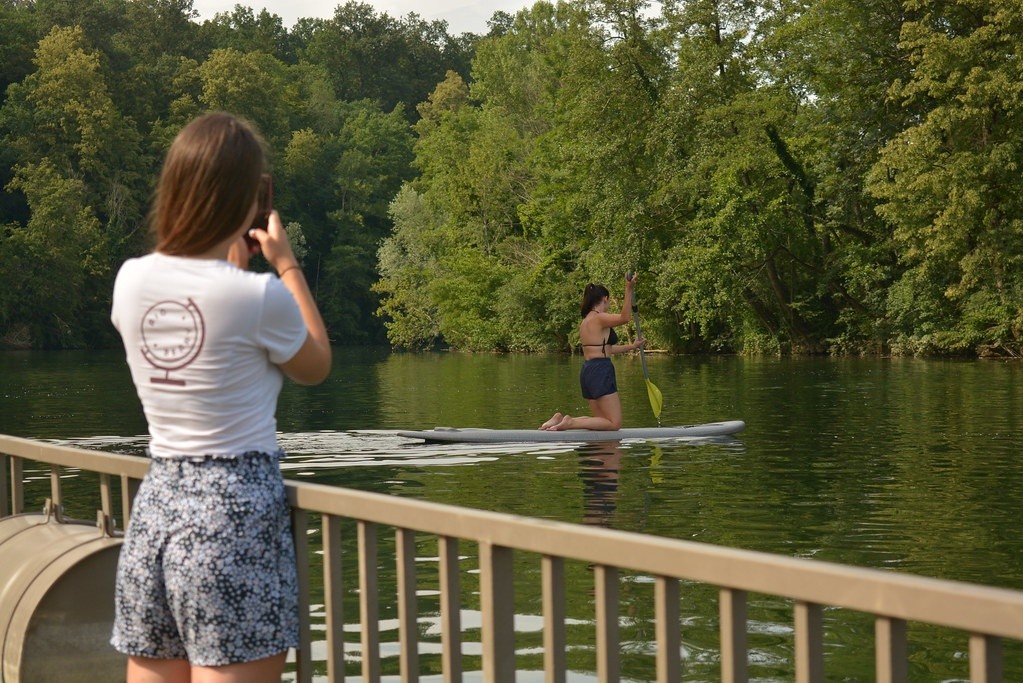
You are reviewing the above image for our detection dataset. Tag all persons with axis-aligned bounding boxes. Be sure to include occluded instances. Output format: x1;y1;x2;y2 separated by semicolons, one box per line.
110;114;333;683
539;272;646;431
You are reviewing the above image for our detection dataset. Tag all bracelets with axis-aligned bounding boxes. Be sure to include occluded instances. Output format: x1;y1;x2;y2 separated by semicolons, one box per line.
279;266;302;279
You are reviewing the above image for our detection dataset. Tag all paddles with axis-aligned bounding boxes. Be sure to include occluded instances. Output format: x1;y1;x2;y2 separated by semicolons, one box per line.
627;269;664;418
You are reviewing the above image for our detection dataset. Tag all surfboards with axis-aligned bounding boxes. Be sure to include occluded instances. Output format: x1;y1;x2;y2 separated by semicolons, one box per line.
394;418;747;443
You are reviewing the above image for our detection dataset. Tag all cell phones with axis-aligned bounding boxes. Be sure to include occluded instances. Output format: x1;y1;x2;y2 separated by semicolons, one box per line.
243;173;273;248
